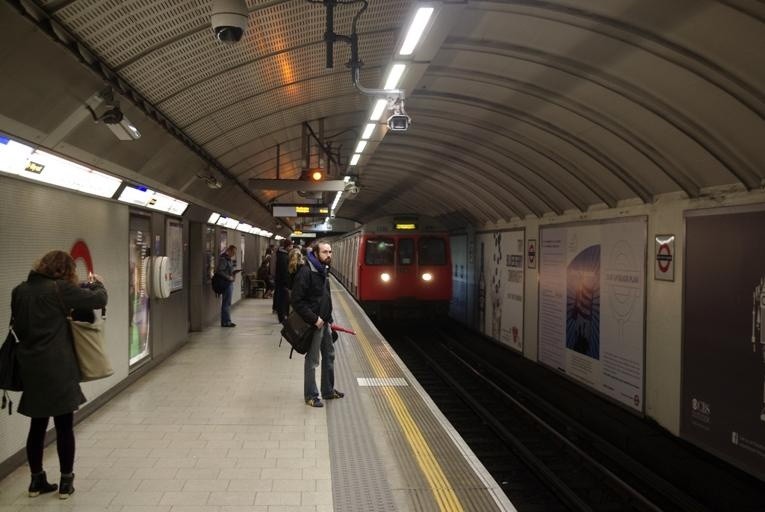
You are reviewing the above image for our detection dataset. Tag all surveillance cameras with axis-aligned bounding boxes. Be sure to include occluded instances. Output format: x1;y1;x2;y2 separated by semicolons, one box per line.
387;112;411;132
210;0;250;44
344;186;360;201
101;109;141;142
207;175;224;191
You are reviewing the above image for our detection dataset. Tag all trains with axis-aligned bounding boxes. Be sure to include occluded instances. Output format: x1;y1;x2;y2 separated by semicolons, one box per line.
323;212;455;330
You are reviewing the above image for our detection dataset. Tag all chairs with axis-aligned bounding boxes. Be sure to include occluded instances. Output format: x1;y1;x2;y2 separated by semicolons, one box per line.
247;274;273;298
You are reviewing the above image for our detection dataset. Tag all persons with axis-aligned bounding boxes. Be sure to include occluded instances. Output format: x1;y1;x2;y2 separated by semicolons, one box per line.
10;249;108;501
217;244;237;327
257;238;345;408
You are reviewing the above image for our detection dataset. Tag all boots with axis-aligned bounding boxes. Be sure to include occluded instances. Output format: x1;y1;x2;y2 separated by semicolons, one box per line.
263;288;272;298
57;474;75;498
29;470;58;496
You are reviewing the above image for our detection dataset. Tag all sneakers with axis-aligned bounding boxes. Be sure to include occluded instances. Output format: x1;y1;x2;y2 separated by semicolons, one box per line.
306;398;322;406
323;389;344;399
221;323;235;327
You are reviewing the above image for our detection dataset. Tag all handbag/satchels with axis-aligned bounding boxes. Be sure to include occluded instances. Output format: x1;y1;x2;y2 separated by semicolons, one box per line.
280;308;314;355
1;330;25;392
68;316;114;379
212;273;230;294
